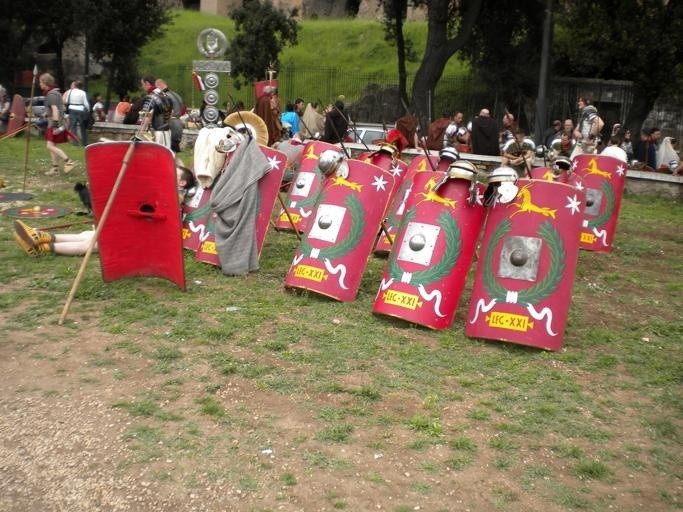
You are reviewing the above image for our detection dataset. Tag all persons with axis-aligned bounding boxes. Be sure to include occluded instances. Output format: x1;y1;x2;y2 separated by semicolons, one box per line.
36;73;79;177
395;94;683;180
0;85;11;135
10;165;195;256
62;75;353;192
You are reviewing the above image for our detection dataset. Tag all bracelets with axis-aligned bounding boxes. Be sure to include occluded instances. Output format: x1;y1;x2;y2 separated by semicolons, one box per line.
51;120;59;128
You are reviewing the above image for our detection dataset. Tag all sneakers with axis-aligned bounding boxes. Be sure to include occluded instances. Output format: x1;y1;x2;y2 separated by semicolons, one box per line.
12;231;41;259
45;166;61;175
64;159;76;175
14;218;41;247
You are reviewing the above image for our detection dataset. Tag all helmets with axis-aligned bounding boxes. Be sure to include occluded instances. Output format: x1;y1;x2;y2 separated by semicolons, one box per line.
215;131;245;153
446;122;459;140
483;165;520;207
368;142;399;165
456;126;467;138
535;145;549;156
434;146;460;170
317;149;344;176
433;159;478;206
669;160;680;172
549;155;574;180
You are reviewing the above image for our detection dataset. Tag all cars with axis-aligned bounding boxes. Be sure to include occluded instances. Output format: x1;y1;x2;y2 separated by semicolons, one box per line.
340;126;389;147
23;96;44;117
